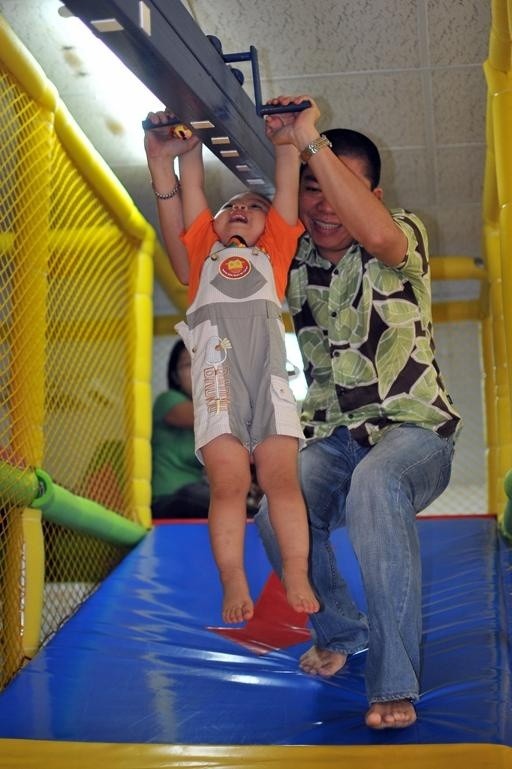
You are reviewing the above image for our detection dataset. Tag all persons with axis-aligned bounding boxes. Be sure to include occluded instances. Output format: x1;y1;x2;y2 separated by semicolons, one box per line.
150;338;264;519
139;88;467;734
138;97;324;626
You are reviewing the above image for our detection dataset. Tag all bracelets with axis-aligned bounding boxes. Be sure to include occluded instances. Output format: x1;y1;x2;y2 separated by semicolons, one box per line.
297;135;332;164
151;175;179;199
